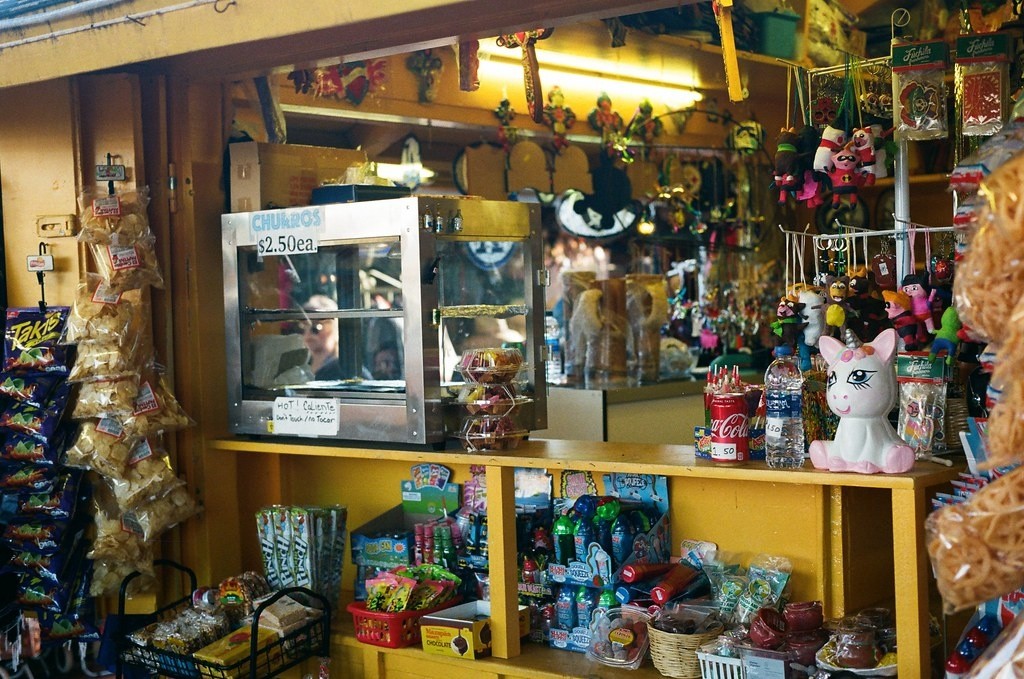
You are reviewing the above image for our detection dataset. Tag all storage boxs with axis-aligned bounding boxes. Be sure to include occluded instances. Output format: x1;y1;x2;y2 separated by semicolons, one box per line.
228;142;369;215
548;472;678;652
194;625;283;679
420;601;529;661
348;464;462;570
751;11;802;59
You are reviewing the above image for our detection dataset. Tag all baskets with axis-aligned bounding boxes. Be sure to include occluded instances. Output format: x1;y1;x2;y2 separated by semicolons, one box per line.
692;646;745;679
345;595;462;649
645;613;723;679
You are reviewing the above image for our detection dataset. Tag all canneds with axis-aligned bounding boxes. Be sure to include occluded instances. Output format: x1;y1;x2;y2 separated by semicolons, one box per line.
710;392;749;466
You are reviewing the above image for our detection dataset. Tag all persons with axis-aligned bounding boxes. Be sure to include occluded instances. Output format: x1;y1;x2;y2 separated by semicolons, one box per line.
291;295;372;381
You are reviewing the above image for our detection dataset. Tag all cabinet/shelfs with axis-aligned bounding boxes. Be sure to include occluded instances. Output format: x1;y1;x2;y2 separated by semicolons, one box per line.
854;74;957;275
212;439;963;679
220;196;547;446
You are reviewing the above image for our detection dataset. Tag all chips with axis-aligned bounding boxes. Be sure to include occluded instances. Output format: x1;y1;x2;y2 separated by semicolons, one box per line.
58;206;200;596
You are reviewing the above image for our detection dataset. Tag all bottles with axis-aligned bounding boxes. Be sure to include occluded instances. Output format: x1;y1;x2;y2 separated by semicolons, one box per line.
946;372;970;449
764;346;805;469
544;310;562;385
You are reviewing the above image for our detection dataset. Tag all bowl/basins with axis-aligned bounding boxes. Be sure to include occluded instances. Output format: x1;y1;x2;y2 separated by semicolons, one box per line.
660;346;698;377
